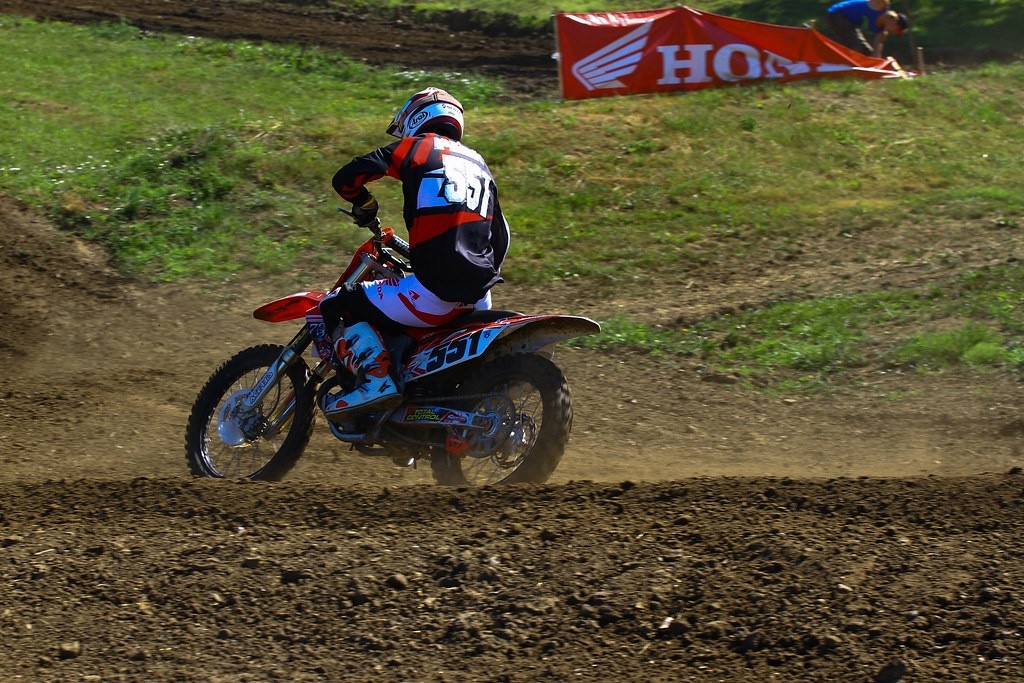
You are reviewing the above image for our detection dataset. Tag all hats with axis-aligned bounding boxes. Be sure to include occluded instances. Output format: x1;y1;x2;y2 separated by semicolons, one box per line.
898;13;908;39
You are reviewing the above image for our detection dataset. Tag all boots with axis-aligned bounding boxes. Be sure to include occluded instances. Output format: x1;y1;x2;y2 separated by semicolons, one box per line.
325;323;403;423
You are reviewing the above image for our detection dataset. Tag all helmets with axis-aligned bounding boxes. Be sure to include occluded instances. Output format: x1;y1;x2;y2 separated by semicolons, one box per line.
386;87;464;139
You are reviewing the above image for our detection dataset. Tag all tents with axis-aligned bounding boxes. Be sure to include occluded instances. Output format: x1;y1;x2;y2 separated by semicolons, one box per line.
553;4;909;97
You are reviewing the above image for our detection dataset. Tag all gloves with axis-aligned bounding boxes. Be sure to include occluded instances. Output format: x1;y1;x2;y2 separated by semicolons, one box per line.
351;191;378;227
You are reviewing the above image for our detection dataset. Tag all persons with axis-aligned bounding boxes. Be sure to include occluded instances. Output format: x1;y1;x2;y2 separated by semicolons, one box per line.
319;87;510;423
826;0;910;57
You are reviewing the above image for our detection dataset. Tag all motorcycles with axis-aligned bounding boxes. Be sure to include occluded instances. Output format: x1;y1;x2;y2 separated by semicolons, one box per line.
181;198;603;488
